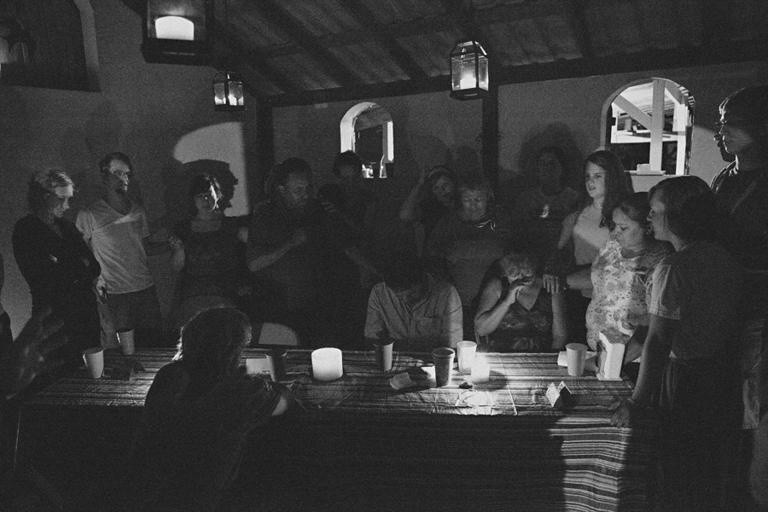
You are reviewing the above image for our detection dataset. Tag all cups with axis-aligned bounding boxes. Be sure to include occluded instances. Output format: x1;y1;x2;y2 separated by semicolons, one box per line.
115;328;137;357
79;346;106;380
372;335;400;373
264;348;291;386
310;347;343;382
565;342;589;377
430;340;490;388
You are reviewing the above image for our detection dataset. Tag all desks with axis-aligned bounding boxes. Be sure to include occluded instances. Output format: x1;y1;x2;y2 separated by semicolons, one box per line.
16;350;666;511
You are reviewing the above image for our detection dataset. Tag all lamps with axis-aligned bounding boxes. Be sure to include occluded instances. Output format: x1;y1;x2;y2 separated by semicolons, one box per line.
449;39;490;100
212;71;246;111
140;0;209;65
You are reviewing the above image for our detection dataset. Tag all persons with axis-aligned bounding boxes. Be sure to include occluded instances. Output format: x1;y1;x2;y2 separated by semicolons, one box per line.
245;145;676;385
165;173;252;347
609;173;750;512
125;306;288;511
0;299;66;403
75;151;172;347
708;85;768;511
12;166;102;361
710;110;736;193
